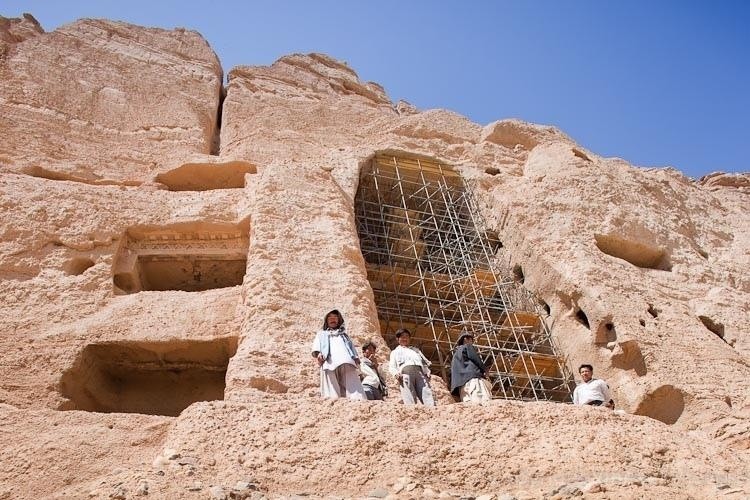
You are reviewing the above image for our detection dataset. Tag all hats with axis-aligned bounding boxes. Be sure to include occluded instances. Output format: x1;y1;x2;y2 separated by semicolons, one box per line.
457;333;473;345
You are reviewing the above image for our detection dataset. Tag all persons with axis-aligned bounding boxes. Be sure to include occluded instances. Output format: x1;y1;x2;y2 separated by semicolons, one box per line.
388;328;436;407
450;332;492;404
573;364;611;409
605;399;627;414
356;342;386;400
310;307;367;399
